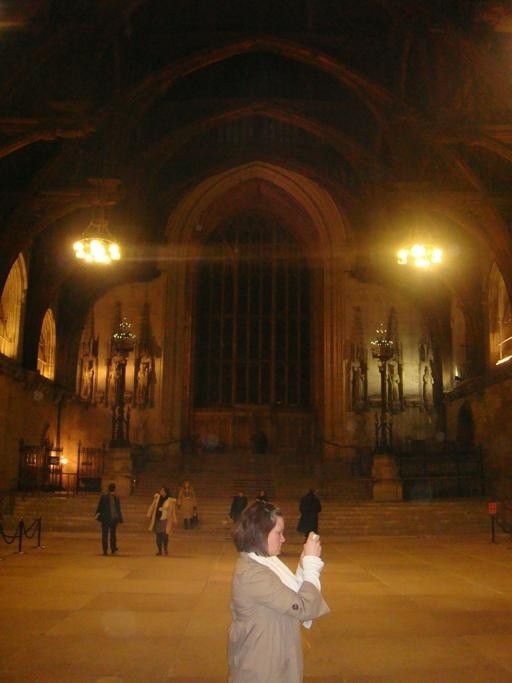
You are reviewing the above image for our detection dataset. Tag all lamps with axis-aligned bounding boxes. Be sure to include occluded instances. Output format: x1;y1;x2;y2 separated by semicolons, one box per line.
71;207;122;266
395;211;445;269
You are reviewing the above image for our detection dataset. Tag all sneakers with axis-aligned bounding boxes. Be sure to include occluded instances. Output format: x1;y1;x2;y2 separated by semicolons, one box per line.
156;551;168;556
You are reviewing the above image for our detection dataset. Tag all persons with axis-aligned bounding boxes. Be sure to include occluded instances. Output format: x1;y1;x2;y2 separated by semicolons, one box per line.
108;363;122;400
134;362;148;403
354;366;365;398
230;489;246;521
146;486;177;556
81;365;93;398
96;483;124;554
228;502;331;683
388;361;401;400
423;363;436;402
297;487;322;537
178;480;198;528
256;489;268;504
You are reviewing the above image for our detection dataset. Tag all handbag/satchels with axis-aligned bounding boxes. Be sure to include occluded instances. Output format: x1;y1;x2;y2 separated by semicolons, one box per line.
193;514;197;524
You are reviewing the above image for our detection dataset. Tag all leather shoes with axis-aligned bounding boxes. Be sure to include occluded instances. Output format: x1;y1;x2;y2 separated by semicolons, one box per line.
103;547;119;555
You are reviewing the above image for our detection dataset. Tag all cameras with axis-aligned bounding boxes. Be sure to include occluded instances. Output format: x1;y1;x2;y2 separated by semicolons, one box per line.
305;531;319;543
